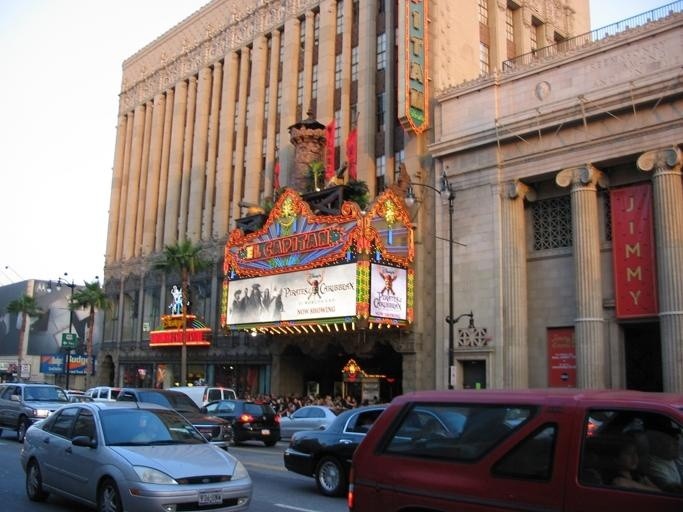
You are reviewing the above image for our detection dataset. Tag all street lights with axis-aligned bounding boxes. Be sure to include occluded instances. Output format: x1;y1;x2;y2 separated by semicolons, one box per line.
404;171;475;389
46;278;76;389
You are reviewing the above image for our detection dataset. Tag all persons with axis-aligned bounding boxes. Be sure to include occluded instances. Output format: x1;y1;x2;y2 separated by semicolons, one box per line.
238;390;389;416
310;280;317;292
128;413;158;441
608;437;661;493
384;275;392;289
231;283;283;321
642;420;682;488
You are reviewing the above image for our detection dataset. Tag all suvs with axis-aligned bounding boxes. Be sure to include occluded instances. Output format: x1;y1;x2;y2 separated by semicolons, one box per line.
0;381;75;443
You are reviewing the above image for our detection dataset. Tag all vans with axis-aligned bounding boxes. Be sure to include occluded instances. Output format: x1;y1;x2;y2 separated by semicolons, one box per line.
348;389;682;512
163;387;237;409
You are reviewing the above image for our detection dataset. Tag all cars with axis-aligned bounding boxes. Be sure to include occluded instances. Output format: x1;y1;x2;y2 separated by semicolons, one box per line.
283;404;466;498
59;386;120;404
201;399;281;447
20;401;253;512
502;410;607;441
278;406;350;441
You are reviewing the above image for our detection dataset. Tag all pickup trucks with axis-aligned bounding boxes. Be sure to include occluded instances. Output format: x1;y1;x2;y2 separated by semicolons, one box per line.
116;388;235;453
